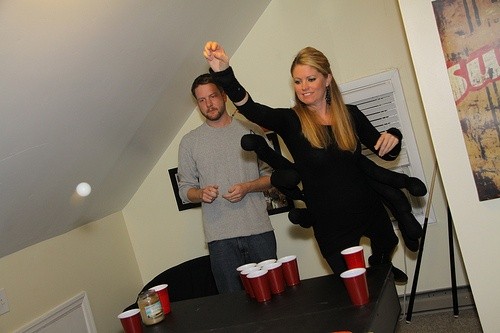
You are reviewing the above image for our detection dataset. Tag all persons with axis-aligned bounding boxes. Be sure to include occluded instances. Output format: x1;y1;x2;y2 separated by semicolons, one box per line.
204;39;408;283
177;73;276;295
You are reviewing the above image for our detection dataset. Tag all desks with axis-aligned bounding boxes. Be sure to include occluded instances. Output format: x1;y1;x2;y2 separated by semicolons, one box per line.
142;263;401;333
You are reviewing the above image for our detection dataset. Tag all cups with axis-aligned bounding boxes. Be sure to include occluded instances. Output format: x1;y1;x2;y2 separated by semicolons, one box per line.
261;263;286;296
148;283;173;317
258;259;277;268
340;267;369;307
240;267;261;299
246;270;272;305
276;255;301;288
118;309;144;333
236;263;257;293
339;246;367;277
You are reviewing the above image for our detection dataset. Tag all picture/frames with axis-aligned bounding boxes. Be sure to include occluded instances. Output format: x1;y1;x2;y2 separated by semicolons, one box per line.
168;167;202;211
256;132;295;216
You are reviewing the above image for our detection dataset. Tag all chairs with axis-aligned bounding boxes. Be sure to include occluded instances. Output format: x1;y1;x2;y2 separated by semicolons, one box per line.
122;255;219;313
